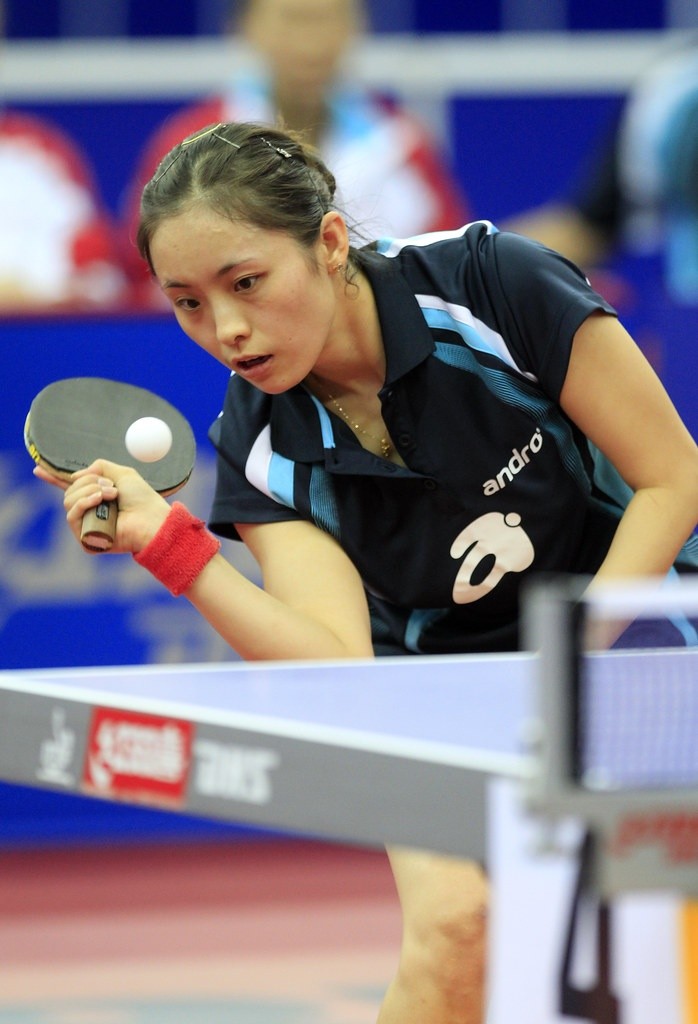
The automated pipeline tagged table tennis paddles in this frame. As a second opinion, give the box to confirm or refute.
[23,376,200,555]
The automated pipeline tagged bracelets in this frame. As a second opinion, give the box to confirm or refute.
[133,499,221,599]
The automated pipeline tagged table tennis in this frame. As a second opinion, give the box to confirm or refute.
[123,414,174,464]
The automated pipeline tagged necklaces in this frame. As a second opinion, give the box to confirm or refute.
[328,390,392,458]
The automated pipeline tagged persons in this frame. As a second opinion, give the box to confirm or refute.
[33,122,697,1023]
[0,0,698,312]
